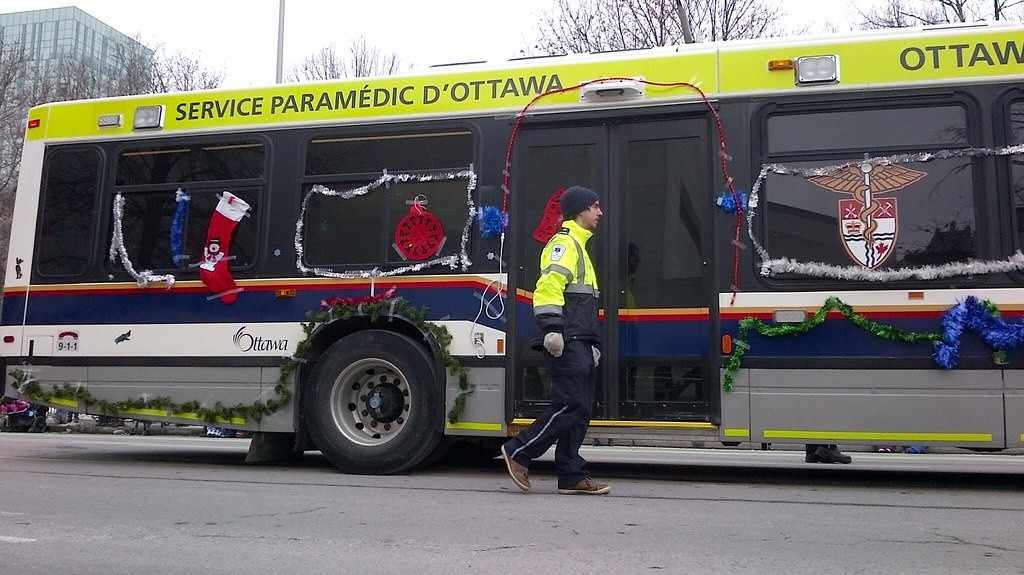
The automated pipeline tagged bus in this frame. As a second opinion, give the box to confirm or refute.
[0,20,1024,476]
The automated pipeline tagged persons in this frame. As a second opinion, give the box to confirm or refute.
[805,443,850,464]
[501,186,612,494]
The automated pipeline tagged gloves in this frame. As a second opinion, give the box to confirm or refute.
[591,345,601,367]
[543,332,564,357]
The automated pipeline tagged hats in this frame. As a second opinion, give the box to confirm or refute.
[559,187,599,221]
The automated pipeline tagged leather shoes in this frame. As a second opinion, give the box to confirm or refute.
[805,450,852,464]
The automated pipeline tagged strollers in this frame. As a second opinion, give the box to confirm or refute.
[0,397,48,434]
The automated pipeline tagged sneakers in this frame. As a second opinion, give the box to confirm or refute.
[501,442,532,490]
[557,478,612,495]
[878,448,891,453]
